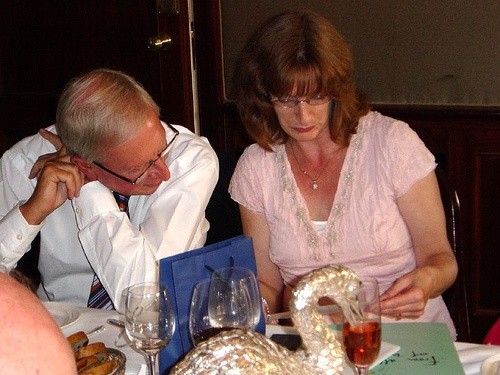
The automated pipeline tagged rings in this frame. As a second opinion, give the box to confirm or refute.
[397,313,403,319]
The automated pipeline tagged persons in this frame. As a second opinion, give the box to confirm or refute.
[0,272,78,375]
[0,70,222,314]
[230,8,461,342]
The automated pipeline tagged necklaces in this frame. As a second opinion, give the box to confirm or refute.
[287,137,332,191]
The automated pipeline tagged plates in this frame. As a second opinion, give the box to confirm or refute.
[481,354,500,375]
[41,300,82,330]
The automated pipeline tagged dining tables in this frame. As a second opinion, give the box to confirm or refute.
[59,307,500,375]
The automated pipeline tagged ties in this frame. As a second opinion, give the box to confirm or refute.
[86,192,131,312]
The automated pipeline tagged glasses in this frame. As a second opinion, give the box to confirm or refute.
[91,116,180,185]
[267,91,334,108]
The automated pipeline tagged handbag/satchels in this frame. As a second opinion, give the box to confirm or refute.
[155,234,266,375]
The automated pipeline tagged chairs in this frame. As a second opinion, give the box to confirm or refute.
[436,168,471,336]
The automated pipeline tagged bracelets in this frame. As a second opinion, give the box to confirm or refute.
[261,295,271,322]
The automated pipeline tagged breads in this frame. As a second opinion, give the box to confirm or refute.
[66,331,116,375]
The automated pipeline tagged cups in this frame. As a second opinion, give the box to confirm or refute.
[207,267,262,329]
[188,277,255,348]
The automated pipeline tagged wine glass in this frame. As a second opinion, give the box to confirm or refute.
[342,276,382,375]
[125,281,177,375]
